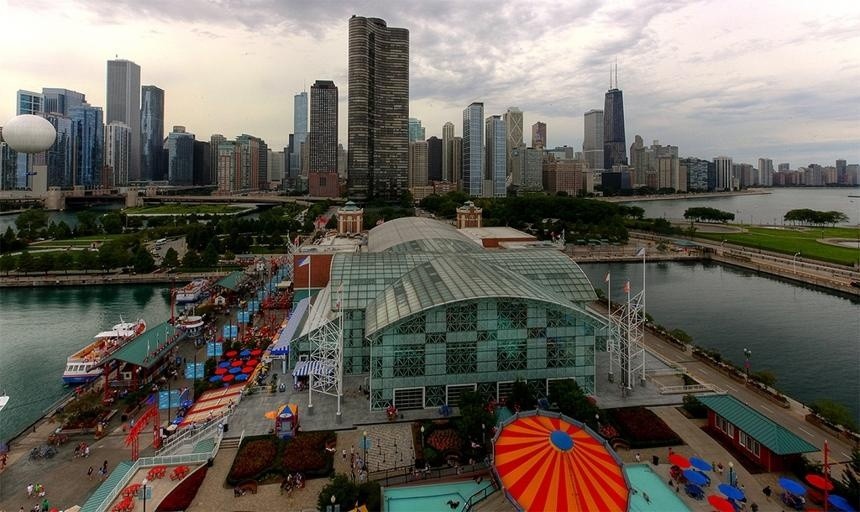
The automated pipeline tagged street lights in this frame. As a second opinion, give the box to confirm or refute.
[721,238,727,254]
[794,252,801,274]
[742,347,751,376]
[420,424,427,446]
[362,430,368,469]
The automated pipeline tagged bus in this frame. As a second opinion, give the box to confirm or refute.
[157,238,166,245]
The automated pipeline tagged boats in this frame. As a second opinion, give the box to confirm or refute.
[62,318,147,385]
[176,279,211,304]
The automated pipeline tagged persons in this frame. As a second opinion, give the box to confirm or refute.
[120,411,137,431]
[712,460,724,475]
[763,486,771,499]
[19,482,51,512]
[739,484,745,495]
[636,453,641,462]
[0,453,7,469]
[74,442,90,458]
[408,464,432,481]
[286,472,303,497]
[798,495,806,504]
[342,441,371,482]
[189,421,196,437]
[171,369,178,380]
[222,413,229,432]
[88,460,108,482]
[750,502,759,512]
